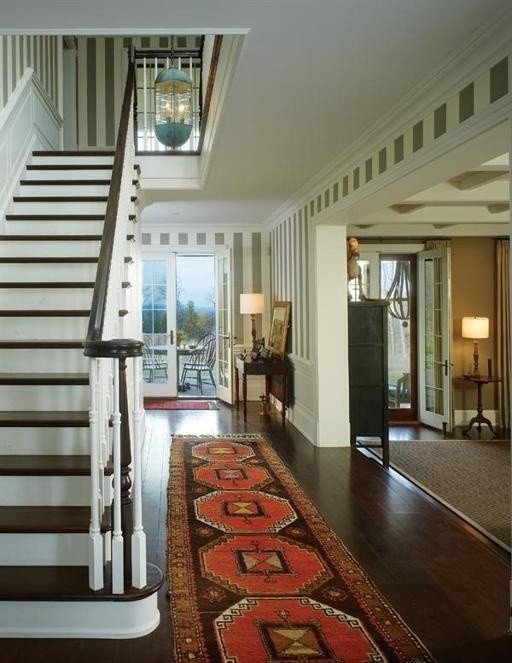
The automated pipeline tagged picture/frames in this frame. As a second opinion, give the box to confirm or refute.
[265,300,292,359]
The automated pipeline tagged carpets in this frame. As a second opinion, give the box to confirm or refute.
[162,430,435,661]
[365,436,510,556]
[142,400,220,411]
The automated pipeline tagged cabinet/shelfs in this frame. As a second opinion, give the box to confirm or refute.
[348,299,393,470]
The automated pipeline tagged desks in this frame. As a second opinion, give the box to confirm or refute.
[455,374,502,438]
[148,344,207,390]
[233,354,290,426]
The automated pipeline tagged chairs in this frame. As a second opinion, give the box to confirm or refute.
[179,331,218,394]
[142,334,168,383]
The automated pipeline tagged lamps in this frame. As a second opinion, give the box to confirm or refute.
[152,35,194,152]
[239,292,264,352]
[461,314,490,378]
